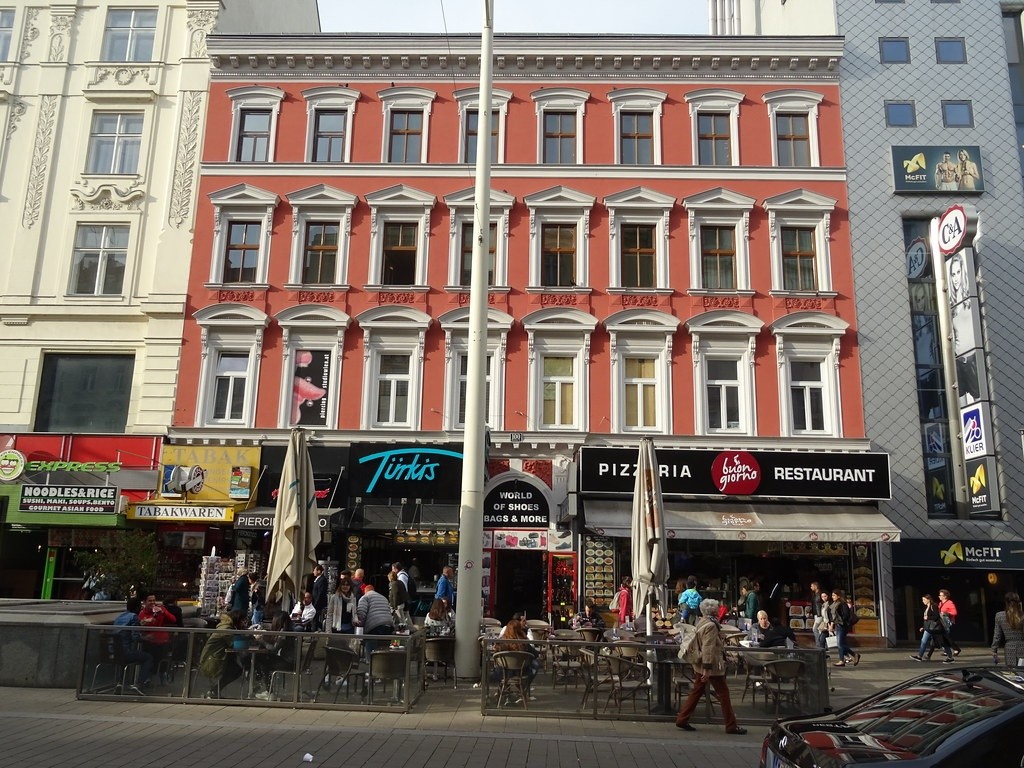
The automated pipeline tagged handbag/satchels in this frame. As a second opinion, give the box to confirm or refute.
[928,621,943,633]
[847,613,859,626]
[940,616,952,632]
[825,631,838,648]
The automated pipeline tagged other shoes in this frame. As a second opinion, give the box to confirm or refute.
[909,655,922,662]
[833,661,845,666]
[676,724,696,731]
[325,674,330,683]
[206,691,222,699]
[853,653,861,666]
[942,659,955,664]
[335,676,350,686]
[727,727,748,735]
[131,684,148,696]
[365,678,381,684]
[940,653,948,656]
[844,656,852,663]
[922,655,930,661]
[113,682,123,695]
[952,648,961,657]
[255,691,274,701]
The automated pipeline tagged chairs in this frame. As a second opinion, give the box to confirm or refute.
[483,618,807,718]
[90,616,457,706]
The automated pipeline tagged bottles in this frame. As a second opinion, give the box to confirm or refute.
[430,621,435,637]
[425,623,430,638]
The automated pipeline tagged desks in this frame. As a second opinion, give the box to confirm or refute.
[374,646,421,702]
[748,652,802,715]
[225,646,269,699]
[487,648,546,697]
[620,635,674,643]
[720,629,748,674]
[640,652,690,715]
[549,637,586,685]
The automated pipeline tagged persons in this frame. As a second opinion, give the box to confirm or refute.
[113,563,1024,735]
[829,590,861,666]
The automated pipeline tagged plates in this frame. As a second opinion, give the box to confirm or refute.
[789,605,804,616]
[805,606,814,618]
[806,619,815,629]
[586,541,614,606]
[789,619,805,629]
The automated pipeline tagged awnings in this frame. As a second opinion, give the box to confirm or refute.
[233,506,344,531]
[583,500,902,541]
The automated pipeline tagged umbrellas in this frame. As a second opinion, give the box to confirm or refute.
[631,433,670,688]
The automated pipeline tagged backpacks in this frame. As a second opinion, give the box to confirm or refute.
[608,590,629,610]
[399,571,417,601]
[677,619,714,664]
